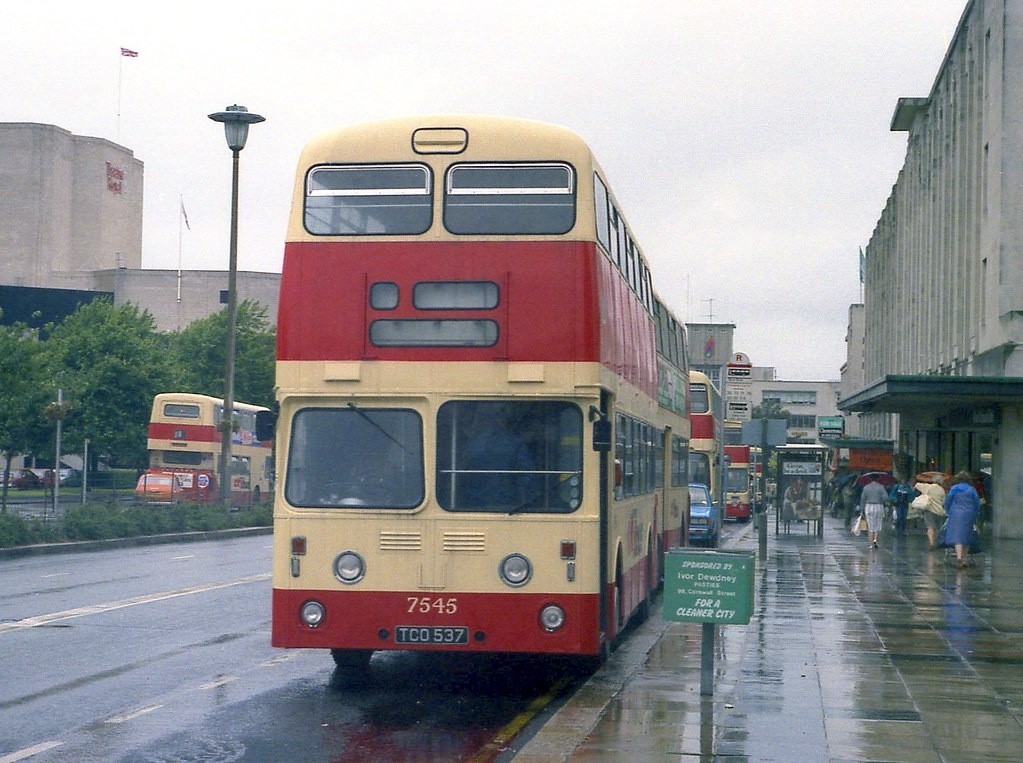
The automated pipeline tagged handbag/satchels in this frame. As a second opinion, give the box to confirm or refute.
[911,485,931,510]
[860,516,868,531]
[893,506,898,519]
[851,515,861,536]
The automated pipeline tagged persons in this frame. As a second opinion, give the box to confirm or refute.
[942,470,979,566]
[842,481,855,531]
[783,477,807,519]
[890,478,915,535]
[909,480,920,497]
[825,483,835,506]
[136,467,144,483]
[859,475,888,551]
[451,414,537,500]
[915,476,946,549]
[975,480,985,534]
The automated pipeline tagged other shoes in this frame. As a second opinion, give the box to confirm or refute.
[928,543,936,551]
[869,546,873,549]
[958,559,963,569]
[963,559,966,567]
[873,540,878,547]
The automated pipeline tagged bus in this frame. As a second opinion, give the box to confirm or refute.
[253,114,692,686]
[145,391,276,512]
[751,446,764,512]
[723,443,751,522]
[688,368,729,547]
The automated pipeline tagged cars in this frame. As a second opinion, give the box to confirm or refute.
[0,468,40,490]
[133,474,185,507]
[29,468,62,488]
[688,482,720,549]
[52,468,84,488]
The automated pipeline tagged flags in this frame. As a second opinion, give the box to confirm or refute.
[121,48,138,57]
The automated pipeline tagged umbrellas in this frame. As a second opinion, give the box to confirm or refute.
[916,472,949,483]
[859,471,896,486]
[837,474,858,491]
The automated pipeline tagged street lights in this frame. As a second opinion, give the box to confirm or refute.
[207,105,266,511]
